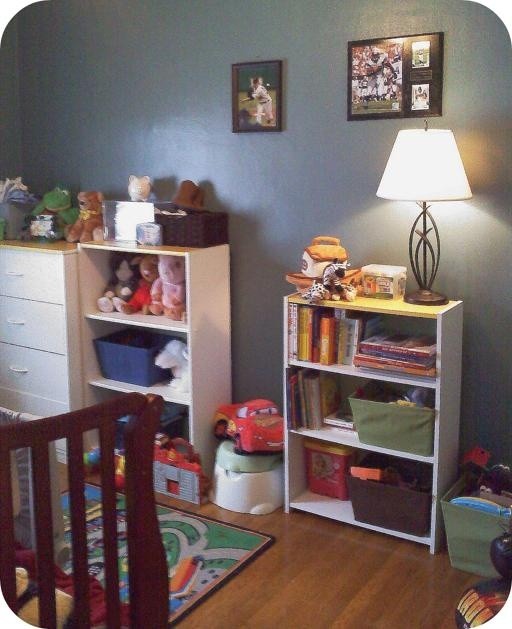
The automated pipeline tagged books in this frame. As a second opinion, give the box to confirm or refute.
[288,302,437,431]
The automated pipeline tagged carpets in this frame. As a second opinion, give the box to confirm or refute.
[43,478,275,623]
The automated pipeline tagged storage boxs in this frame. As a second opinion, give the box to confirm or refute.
[87,329,178,387]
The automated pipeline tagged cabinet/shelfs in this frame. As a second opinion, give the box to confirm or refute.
[76,237,232,484]
[1,248,93,464]
[280,288,466,555]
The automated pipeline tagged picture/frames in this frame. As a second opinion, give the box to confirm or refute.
[230,60,282,132]
[347,31,445,118]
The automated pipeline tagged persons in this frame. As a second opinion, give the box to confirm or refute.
[351,46,402,106]
[238,77,274,123]
[414,86,427,99]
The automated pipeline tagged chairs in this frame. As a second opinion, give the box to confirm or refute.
[1,391,174,629]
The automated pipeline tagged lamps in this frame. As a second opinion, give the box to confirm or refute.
[374,120,475,305]
